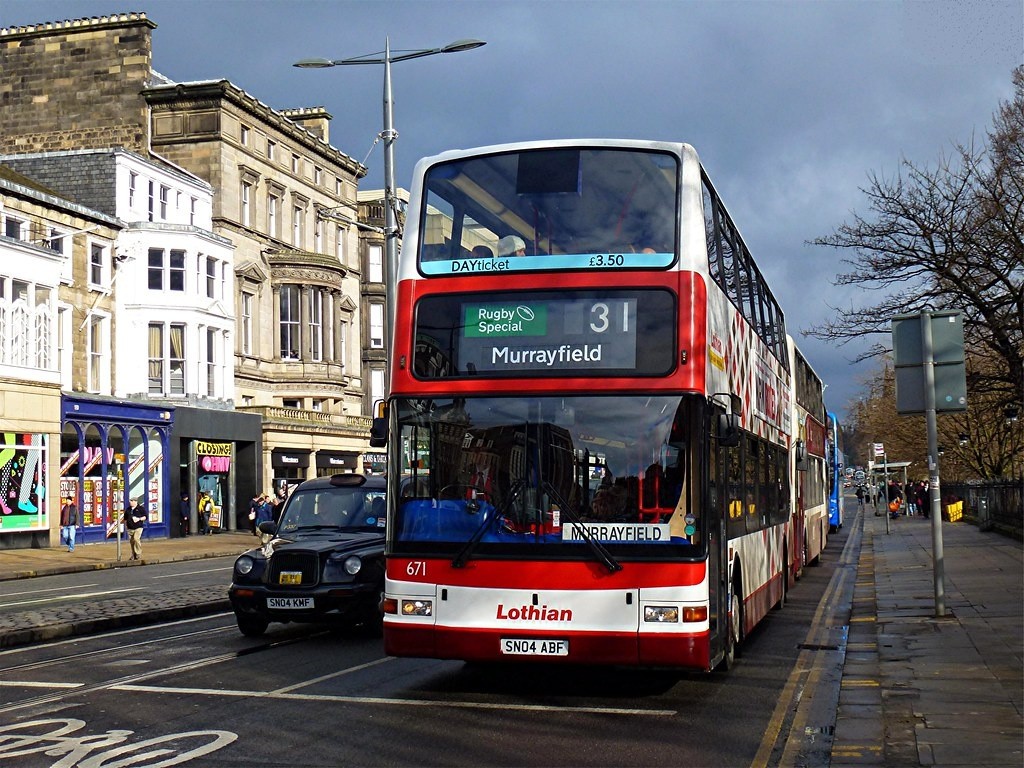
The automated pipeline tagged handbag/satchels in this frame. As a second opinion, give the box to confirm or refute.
[249,508,256,520]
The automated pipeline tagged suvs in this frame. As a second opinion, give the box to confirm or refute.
[229,472,389,639]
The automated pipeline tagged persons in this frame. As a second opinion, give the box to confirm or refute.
[197,490,215,536]
[855,478,931,519]
[578,463,678,523]
[495,235,530,258]
[179,494,191,537]
[60,495,80,554]
[312,494,344,530]
[364,495,387,530]
[456,446,511,509]
[249,491,274,538]
[123,497,149,561]
[470,244,495,259]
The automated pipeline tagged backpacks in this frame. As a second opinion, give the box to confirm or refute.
[203,497,211,512]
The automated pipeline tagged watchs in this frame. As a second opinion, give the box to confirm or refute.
[139,517,142,522]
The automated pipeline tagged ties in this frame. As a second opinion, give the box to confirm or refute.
[476,471,485,501]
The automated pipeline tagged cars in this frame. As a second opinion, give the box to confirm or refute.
[845,480,851,486]
[853,481,861,487]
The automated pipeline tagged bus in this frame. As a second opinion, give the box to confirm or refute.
[369,138,846,679]
[855,471,865,480]
[846,468,853,478]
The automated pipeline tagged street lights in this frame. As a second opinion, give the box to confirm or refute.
[292,39,487,492]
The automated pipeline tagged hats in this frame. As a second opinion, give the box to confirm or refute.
[130,496,137,501]
[66,496,72,501]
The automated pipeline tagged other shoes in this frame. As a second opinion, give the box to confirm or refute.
[923,514,930,519]
[68,550,74,552]
[910,512,913,516]
[209,530,213,536]
[130,554,135,561]
[205,532,208,535]
[135,554,142,560]
